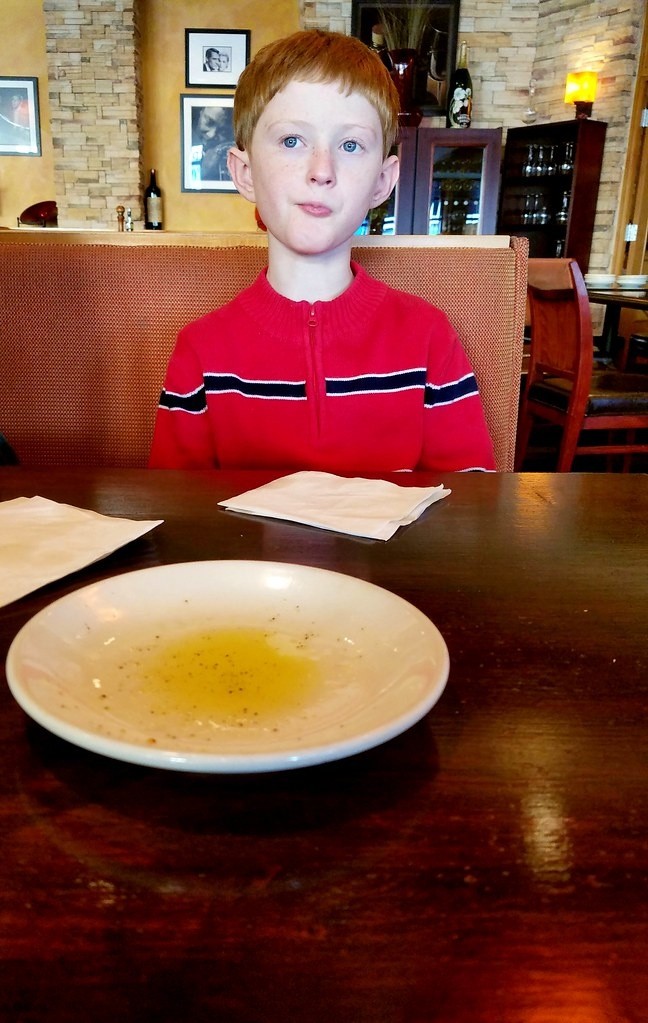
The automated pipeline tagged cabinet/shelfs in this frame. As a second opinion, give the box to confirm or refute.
[358,129,501,235]
[496,120,608,280]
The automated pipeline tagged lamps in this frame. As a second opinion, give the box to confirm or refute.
[565,72,598,119]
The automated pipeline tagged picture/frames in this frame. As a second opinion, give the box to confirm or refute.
[180,93,239,193]
[351,0,459,128]
[185,28,250,89]
[0,75,41,157]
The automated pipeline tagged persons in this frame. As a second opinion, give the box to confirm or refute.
[219,53,229,72]
[145,30,499,475]
[10,94,27,129]
[203,47,219,72]
[195,107,232,151]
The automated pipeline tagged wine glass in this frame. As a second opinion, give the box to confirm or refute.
[515,141,576,260]
[356,142,481,232]
[420,8,449,106]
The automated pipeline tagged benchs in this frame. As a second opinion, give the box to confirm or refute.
[1,230,529,469]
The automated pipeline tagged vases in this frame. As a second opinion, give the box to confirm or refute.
[387,49,422,127]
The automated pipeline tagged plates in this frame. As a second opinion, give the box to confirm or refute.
[584,272,648,287]
[7,559,449,773]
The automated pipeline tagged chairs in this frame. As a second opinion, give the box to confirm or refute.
[512,259,647,474]
[626,330,647,372]
[522,344,607,374]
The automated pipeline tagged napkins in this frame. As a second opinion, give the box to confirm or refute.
[217,469,452,541]
[1,496,163,607]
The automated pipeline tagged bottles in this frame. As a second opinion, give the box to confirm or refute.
[125,208,134,231]
[369,25,387,62]
[144,168,163,230]
[444,41,474,128]
[116,206,124,232]
[520,78,539,123]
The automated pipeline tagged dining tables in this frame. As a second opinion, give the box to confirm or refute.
[0,472,648,1021]
[585,274,648,355]
